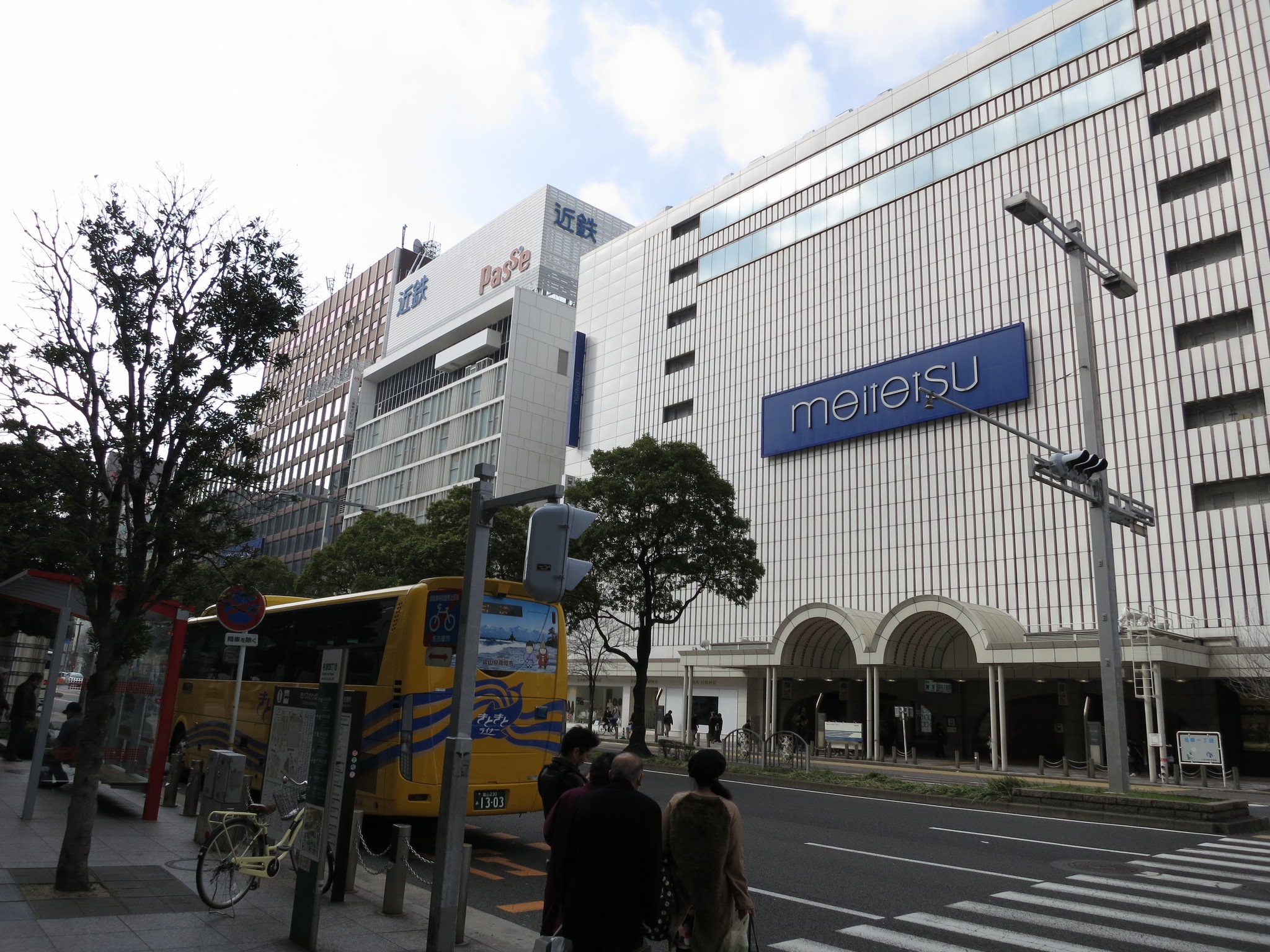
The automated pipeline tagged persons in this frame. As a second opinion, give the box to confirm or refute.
[42,701,81,787]
[6,672,44,761]
[538,706,756,952]
[606,699,613,711]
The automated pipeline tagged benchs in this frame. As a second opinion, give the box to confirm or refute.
[63,758,149,790]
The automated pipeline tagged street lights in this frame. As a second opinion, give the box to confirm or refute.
[1006,190,1138,794]
[276,487,379,549]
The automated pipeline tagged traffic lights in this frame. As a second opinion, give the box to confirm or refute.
[1048,449,1108,483]
[523,503,599,604]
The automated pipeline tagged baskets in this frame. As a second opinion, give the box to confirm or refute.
[272,782,307,820]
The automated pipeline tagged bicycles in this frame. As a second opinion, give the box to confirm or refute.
[195,773,335,919]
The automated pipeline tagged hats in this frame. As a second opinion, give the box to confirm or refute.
[0,666,10,673]
[62,702,82,714]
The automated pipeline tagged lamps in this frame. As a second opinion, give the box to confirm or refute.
[1057,628,1071,631]
[1118,607,1170,630]
[700,640,710,651]
[741,639,750,642]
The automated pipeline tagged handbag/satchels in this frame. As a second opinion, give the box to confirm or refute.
[532,923,572,952]
[720,912,750,952]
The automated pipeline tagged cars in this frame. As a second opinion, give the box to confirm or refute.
[40,668,68,689]
[64,672,84,687]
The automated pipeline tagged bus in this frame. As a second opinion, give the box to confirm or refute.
[168,578,570,856]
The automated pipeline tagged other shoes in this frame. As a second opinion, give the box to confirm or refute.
[52,780,69,787]
[7,756,22,761]
